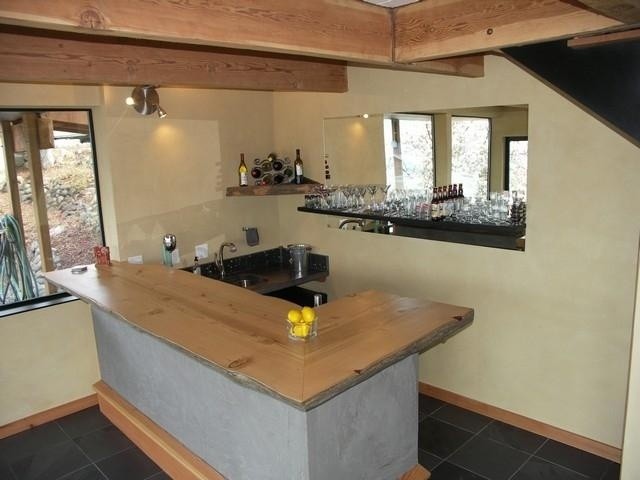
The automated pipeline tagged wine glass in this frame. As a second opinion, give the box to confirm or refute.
[465,191,525,224]
[341,184,434,218]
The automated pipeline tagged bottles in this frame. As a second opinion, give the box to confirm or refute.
[294,148,304,184]
[430,183,464,221]
[239,153,248,187]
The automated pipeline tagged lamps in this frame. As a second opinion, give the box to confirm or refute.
[125,85,168,119]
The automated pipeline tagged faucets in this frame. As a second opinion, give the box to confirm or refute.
[338,218,368,231]
[212,241,238,279]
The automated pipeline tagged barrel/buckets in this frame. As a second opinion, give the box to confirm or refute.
[287,242,312,272]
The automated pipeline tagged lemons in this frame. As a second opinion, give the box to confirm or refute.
[287,306,315,338]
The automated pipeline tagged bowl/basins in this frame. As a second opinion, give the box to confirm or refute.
[287,319,319,341]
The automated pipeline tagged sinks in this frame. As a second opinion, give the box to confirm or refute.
[222,275,265,289]
